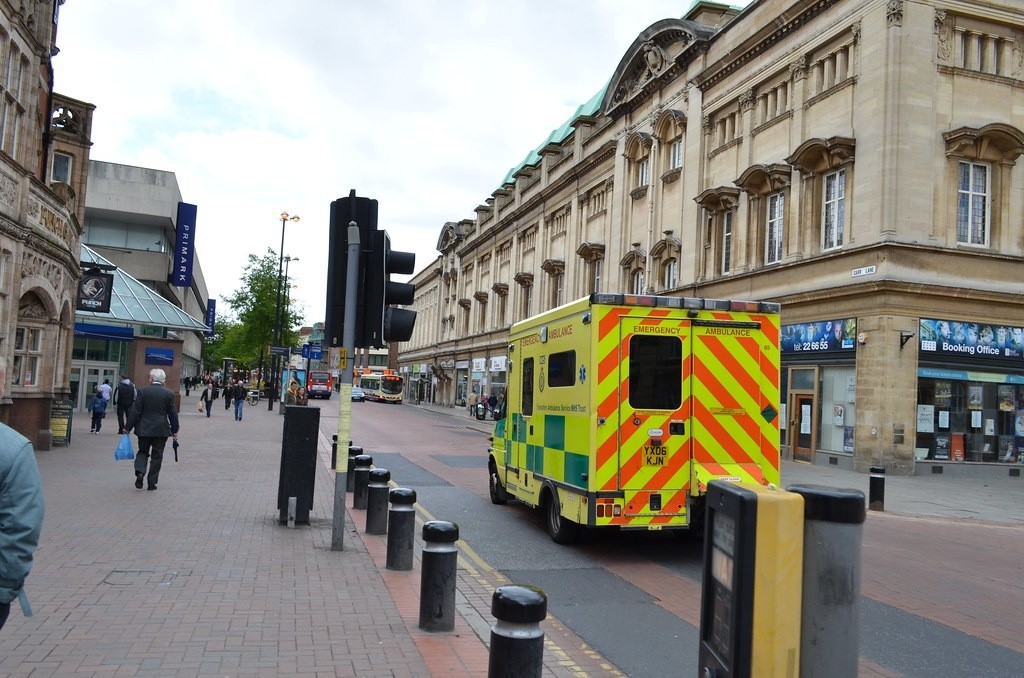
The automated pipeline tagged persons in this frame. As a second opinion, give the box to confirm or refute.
[834,323,844,340]
[233,380,247,421]
[284,371,301,391]
[123,368,180,490]
[98,378,112,418]
[200,383,217,418]
[296,386,309,406]
[88,388,108,434]
[0,423,45,628]
[488,393,498,417]
[112,372,137,434]
[214,382,221,398]
[222,382,233,409]
[284,381,297,412]
[481,393,488,412]
[497,393,504,413]
[185,378,194,396]
[469,390,478,416]
[200,375,203,386]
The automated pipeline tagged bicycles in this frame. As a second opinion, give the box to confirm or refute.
[231,389,259,406]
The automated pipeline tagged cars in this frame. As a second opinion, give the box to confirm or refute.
[351,386,366,402]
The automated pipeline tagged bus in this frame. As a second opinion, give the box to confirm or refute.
[359,368,406,405]
[306,370,334,400]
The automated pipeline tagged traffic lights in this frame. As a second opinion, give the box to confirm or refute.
[373,228,418,351]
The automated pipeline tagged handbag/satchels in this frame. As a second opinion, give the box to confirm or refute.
[198,401,204,412]
[114,433,135,461]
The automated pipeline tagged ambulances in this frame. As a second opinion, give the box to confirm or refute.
[473,291,783,546]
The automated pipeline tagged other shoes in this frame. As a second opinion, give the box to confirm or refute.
[135,473,143,489]
[148,484,157,490]
[90,428,95,433]
[239,418,242,421]
[207,415,210,417]
[235,417,238,420]
[95,432,100,435]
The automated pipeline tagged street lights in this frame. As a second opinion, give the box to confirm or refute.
[267,211,301,411]
